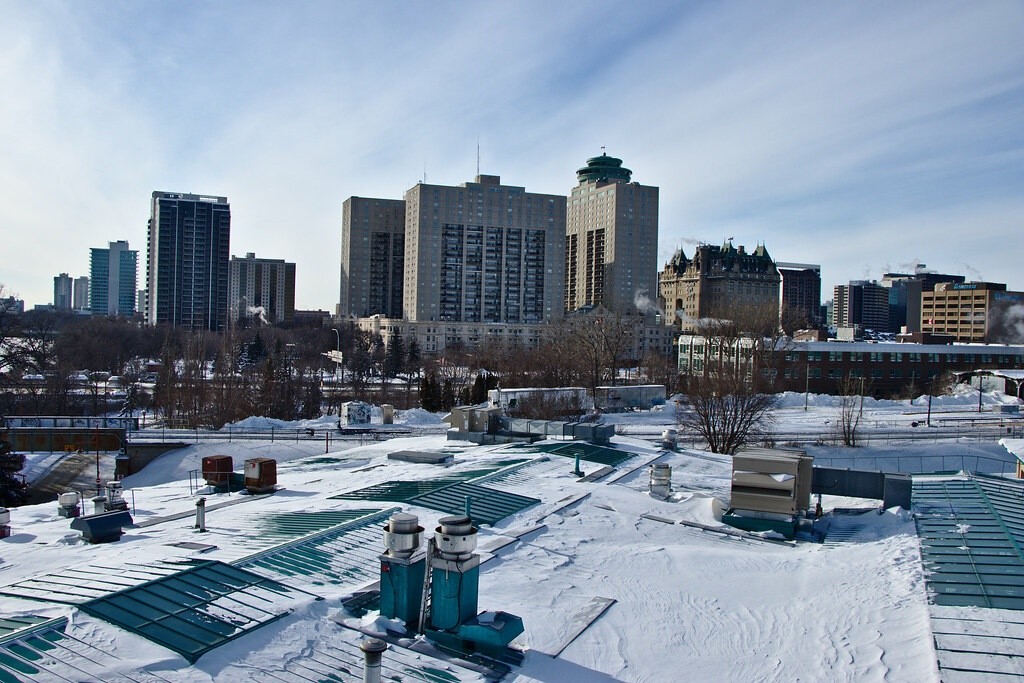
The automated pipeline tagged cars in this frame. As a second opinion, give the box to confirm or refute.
[22,369,157,383]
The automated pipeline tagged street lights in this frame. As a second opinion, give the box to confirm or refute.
[332,327,339,393]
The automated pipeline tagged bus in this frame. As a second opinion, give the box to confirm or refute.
[486,387,588,415]
[595,384,667,413]
